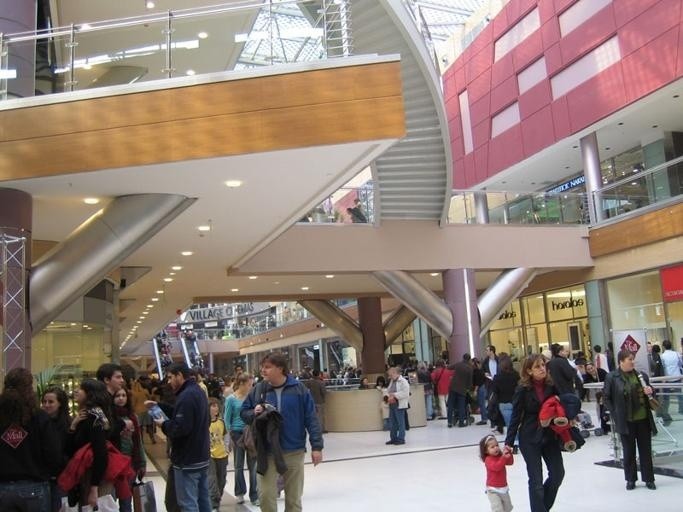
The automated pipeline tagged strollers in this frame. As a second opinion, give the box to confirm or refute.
[574,409,605,439]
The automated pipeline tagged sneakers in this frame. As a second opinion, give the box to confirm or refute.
[393,441,405,445]
[213,507,219,512]
[646,481,656,490]
[385,440,395,445]
[236,494,244,504]
[251,498,261,506]
[426,413,489,428]
[626,480,635,490]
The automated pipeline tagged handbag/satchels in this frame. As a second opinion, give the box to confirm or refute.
[81,493,121,512]
[237,381,267,459]
[636,370,661,411]
[132,478,157,512]
[434,385,438,397]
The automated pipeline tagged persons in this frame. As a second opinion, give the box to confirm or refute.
[352,199,365,224]
